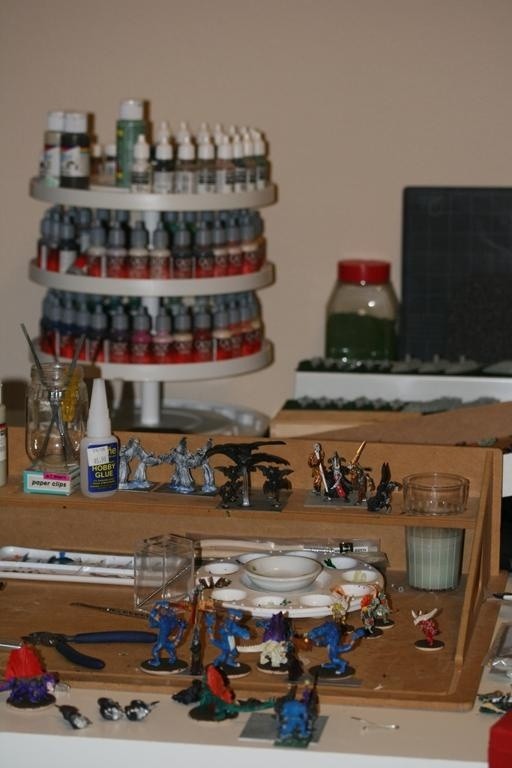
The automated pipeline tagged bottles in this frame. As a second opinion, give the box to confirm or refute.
[1,384,8,487]
[324,259,399,359]
[39,288,262,363]
[78,377,120,498]
[41,110,62,183]
[58,111,91,189]
[38,204,267,279]
[93,97,271,193]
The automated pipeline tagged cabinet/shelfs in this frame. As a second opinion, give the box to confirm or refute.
[0,407,502,713]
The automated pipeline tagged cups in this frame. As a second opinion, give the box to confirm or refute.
[135,533,194,614]
[24,361,89,471]
[403,472,470,592]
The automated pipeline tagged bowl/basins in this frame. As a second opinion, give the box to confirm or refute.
[241,556,323,590]
